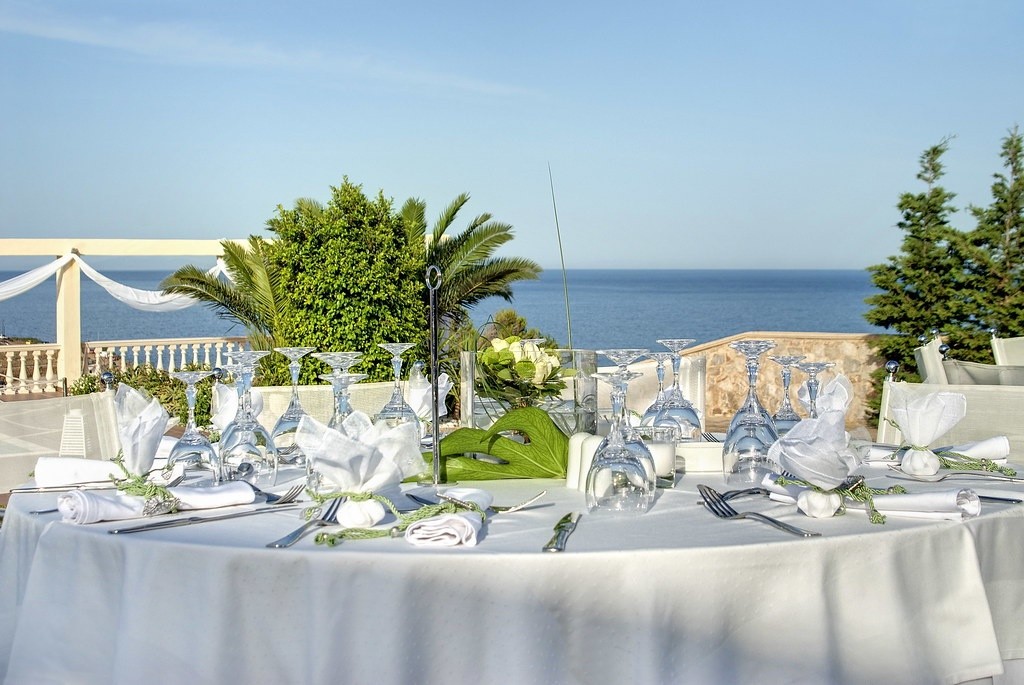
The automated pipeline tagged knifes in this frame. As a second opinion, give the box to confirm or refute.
[542,511,581,552]
[107,504,296,533]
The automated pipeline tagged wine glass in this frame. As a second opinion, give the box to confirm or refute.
[584,337,836,517]
[167,370,221,487]
[222,346,369,487]
[375,343,421,447]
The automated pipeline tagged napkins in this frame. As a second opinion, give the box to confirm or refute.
[32,377,1011,551]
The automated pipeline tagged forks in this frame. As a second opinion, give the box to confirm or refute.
[243,482,306,505]
[488,489,547,514]
[699,488,822,538]
[885,464,1024,484]
[265,497,348,547]
[165,475,186,486]
[701,432,725,442]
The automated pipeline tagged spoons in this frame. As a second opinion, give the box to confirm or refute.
[406,493,436,507]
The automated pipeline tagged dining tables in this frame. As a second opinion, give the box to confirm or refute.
[0,467,1024,685]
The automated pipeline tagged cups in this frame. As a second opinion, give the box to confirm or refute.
[634,426,676,488]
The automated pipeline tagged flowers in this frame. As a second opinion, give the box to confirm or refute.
[470,318,568,445]
